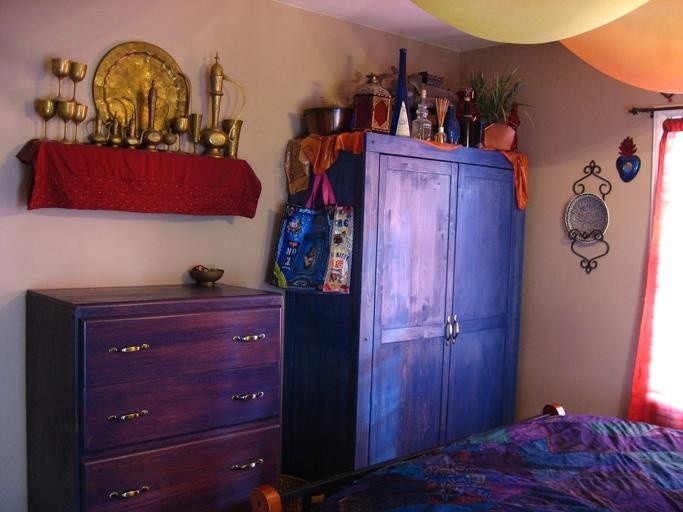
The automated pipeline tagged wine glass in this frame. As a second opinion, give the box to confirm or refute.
[36,57,87,144]
[189,113,203,155]
[172,118,189,154]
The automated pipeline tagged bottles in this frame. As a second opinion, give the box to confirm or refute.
[461,96,473,148]
[445,105,460,145]
[509,102,520,151]
[411,90,433,143]
[434,127,448,144]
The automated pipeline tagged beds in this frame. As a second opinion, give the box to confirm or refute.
[250,403,683,512]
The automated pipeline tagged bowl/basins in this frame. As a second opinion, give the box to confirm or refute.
[303,107,352,136]
[189,270,223,287]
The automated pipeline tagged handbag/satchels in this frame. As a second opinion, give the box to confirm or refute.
[269,172,354,295]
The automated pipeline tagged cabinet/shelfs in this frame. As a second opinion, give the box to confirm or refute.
[282,132,528,477]
[27,283,284,511]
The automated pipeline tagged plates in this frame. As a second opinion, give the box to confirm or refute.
[91,41,192,130]
[565,192,608,245]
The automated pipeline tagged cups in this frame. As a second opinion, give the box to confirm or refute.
[222,119,243,159]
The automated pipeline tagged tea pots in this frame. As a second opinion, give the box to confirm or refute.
[85,107,147,149]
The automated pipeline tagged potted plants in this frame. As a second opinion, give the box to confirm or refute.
[471,63,539,151]
